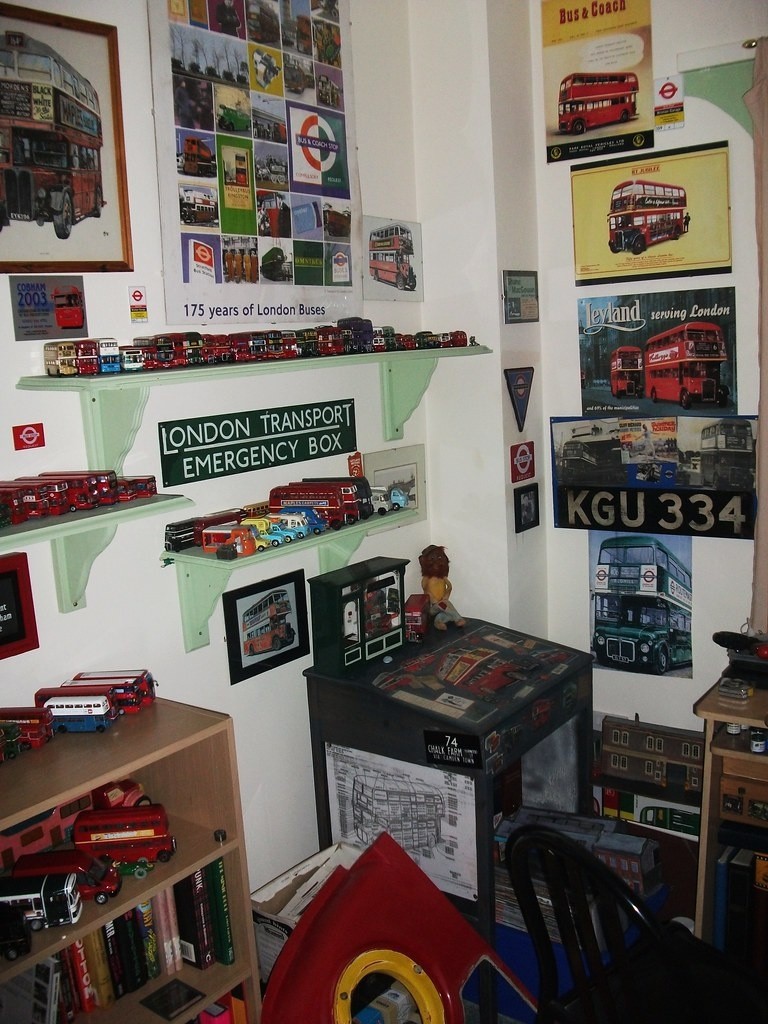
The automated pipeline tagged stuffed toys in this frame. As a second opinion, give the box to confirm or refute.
[418,544,466,630]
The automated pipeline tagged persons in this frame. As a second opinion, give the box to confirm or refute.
[216,0,241,38]
[225,247,258,284]
[637,423,659,457]
[174,79,214,131]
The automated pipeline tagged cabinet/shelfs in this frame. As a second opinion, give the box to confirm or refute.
[0,344,493,652]
[0,698,264,1024]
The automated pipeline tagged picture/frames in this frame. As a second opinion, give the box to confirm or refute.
[513,483,540,533]
[363,444,427,537]
[221,568,311,686]
[0,551,40,661]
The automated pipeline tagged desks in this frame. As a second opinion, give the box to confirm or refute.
[694,677,768,946]
[304,615,596,1024]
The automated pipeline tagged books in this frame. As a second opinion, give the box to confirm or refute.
[713,845,768,982]
[0,858,237,1024]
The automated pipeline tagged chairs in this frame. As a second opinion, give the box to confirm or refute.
[504,824,768,1024]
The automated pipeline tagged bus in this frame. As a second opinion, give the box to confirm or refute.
[606,180,691,255]
[698,418,757,492]
[592,535,693,677]
[562,439,603,487]
[610,346,644,400]
[402,593,432,649]
[0,669,160,766]
[557,72,640,136]
[368,224,418,292]
[242,588,297,658]
[0,469,157,528]
[644,321,730,410]
[1,772,177,960]
[162,476,409,559]
[0,26,99,241]
[43,314,467,378]
[173,3,355,290]
